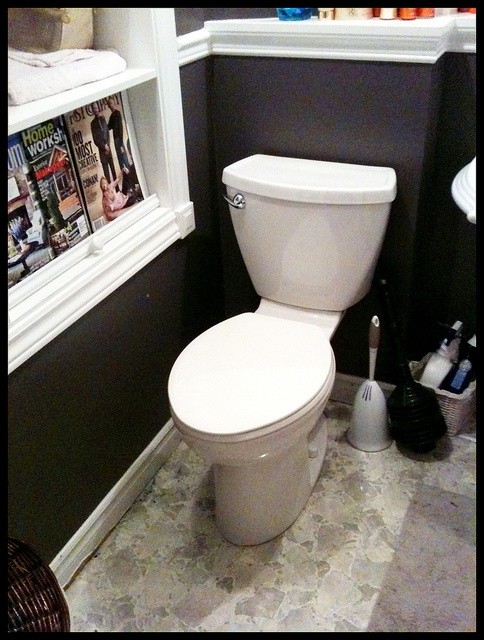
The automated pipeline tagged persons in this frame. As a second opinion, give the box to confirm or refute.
[89,101,119,193]
[106,96,130,171]
[121,137,143,201]
[98,168,142,212]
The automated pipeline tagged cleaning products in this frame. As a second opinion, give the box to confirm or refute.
[443,336,477,395]
[417,321,464,387]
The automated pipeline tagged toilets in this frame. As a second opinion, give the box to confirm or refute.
[167,152,399,547]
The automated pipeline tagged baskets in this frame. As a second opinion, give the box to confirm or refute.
[411,352,476,436]
[6,537,71,632]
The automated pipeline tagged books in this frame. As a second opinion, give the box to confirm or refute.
[19,117,91,256]
[63,92,146,235]
[8,133,56,288]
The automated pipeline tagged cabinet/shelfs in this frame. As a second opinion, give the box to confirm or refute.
[8,9,196,375]
